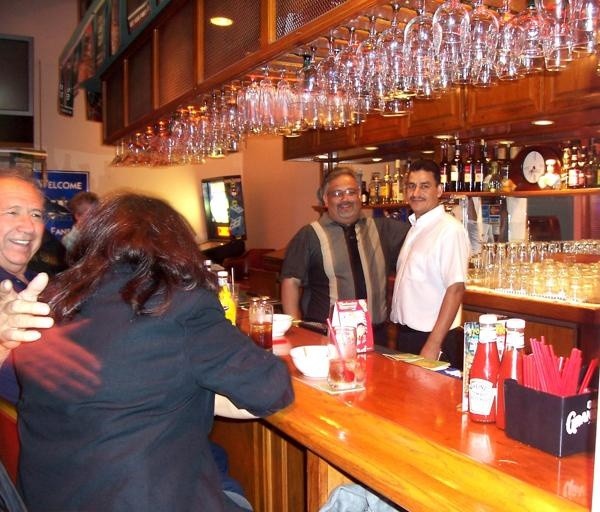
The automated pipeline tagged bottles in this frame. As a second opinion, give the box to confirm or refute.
[249,296,270,324]
[466,240,600,303]
[204,259,212,271]
[217,272,228,288]
[495,318,528,432]
[487,197,504,241]
[534,159,560,190]
[438,140,525,192]
[361,159,411,206]
[467,312,500,422]
[560,138,600,189]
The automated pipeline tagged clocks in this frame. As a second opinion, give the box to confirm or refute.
[507,146,563,192]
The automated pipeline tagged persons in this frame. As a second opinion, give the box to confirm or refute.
[61,192,98,253]
[390,159,469,371]
[16,194,294,512]
[0,167,54,485]
[282,168,410,349]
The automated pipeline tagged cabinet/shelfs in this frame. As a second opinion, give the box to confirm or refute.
[462,305,580,362]
[284,38,356,163]
[467,29,600,139]
[358,47,466,151]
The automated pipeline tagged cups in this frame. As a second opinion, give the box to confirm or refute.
[329,325,357,389]
[223,283,240,302]
[247,304,273,353]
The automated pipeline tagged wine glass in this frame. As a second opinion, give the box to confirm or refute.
[106,0,600,168]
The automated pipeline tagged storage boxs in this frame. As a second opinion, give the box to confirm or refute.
[502,369,597,457]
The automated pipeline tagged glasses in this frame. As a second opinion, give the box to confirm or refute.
[332,190,358,197]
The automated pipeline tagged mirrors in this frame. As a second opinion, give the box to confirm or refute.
[464,195,600,255]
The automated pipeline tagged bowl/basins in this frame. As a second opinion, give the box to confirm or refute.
[272,314,292,336]
[289,344,329,377]
